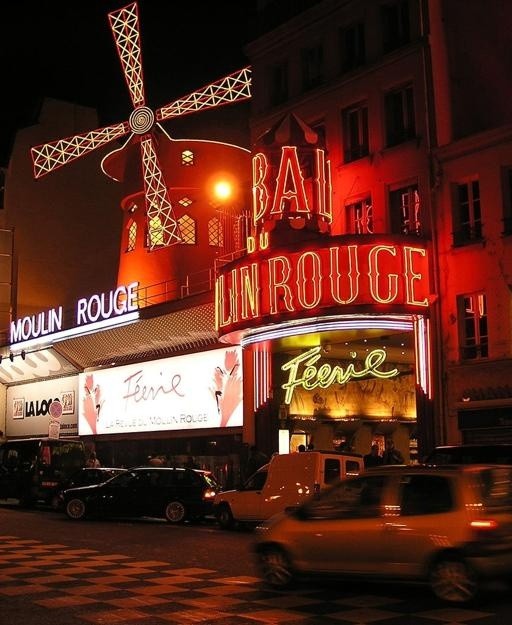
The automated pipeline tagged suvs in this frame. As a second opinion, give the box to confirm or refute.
[64,467,220,525]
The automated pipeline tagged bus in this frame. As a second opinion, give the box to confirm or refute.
[1,438,85,506]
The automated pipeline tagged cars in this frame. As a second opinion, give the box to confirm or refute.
[250,464,511,605]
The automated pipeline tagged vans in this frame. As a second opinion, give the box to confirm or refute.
[213,451,363,531]
[70,467,139,488]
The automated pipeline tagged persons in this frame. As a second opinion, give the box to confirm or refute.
[147,452,201,470]
[383,438,405,464]
[86,451,101,468]
[364,444,382,468]
